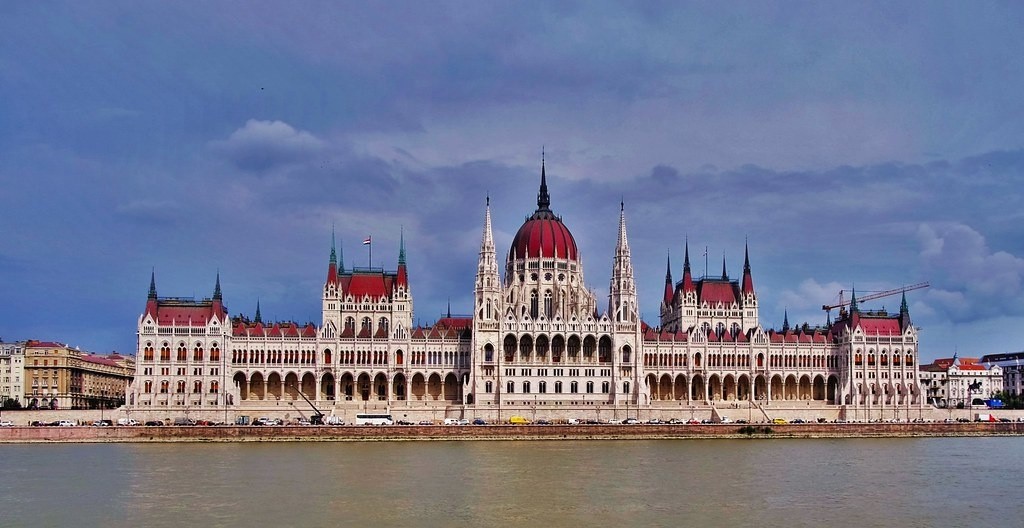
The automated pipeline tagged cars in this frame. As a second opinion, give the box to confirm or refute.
[93,420,108,426]
[773,418,788,424]
[154,421,163,426]
[419,420,434,425]
[0,420,14,426]
[538,416,749,425]
[31,421,43,427]
[793,414,1024,423]
[299,420,311,426]
[145,421,155,426]
[59,420,77,426]
[444,418,488,425]
[258,417,282,426]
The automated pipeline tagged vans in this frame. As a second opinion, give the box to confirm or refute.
[510,416,530,425]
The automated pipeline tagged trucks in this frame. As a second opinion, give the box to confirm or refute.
[118,418,138,425]
[174,416,195,426]
[327,416,345,425]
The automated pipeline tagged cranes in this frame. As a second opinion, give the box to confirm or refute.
[822,281,930,318]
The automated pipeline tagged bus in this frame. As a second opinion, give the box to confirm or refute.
[356,414,394,425]
